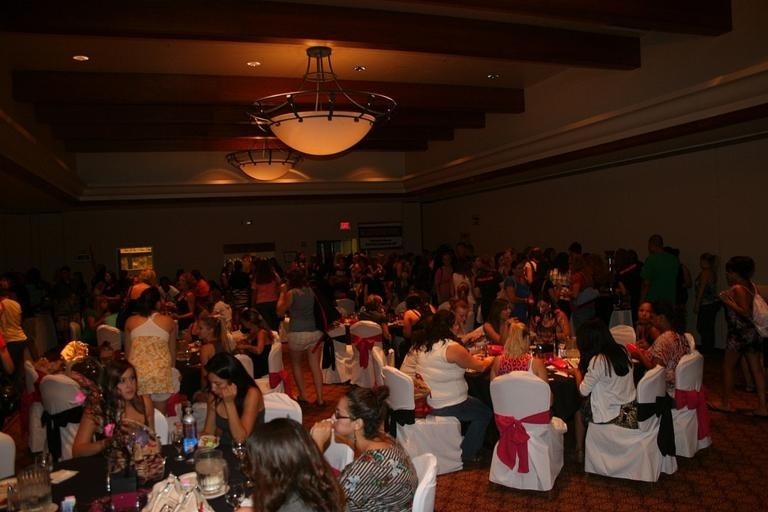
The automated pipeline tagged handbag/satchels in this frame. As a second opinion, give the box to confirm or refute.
[497,288,517,302]
[574,288,600,306]
[120,419,164,483]
[141,473,213,512]
[614,404,638,428]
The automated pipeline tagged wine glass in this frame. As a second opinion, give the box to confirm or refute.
[225,479,246,511]
[183,348,192,366]
[171,428,186,463]
[231,436,246,470]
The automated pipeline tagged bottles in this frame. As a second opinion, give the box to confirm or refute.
[16,470,52,511]
[183,408,198,438]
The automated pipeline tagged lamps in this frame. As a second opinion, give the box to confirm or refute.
[225,104,305,182]
[246,46,399,155]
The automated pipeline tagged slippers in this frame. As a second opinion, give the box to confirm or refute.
[317,400,325,407]
[743,410,768,418]
[706,400,729,411]
[298,396,307,402]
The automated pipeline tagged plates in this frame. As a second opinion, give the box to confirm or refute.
[196,482,230,500]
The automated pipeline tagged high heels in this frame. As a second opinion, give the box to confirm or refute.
[574,449,583,462]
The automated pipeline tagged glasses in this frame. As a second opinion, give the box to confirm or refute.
[335,409,350,419]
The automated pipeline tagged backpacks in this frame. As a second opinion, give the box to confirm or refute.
[745,283,768,338]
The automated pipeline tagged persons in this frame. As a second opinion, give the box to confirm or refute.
[1,231,767,512]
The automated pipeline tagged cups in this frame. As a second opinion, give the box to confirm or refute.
[37,453,51,469]
[194,447,228,494]
